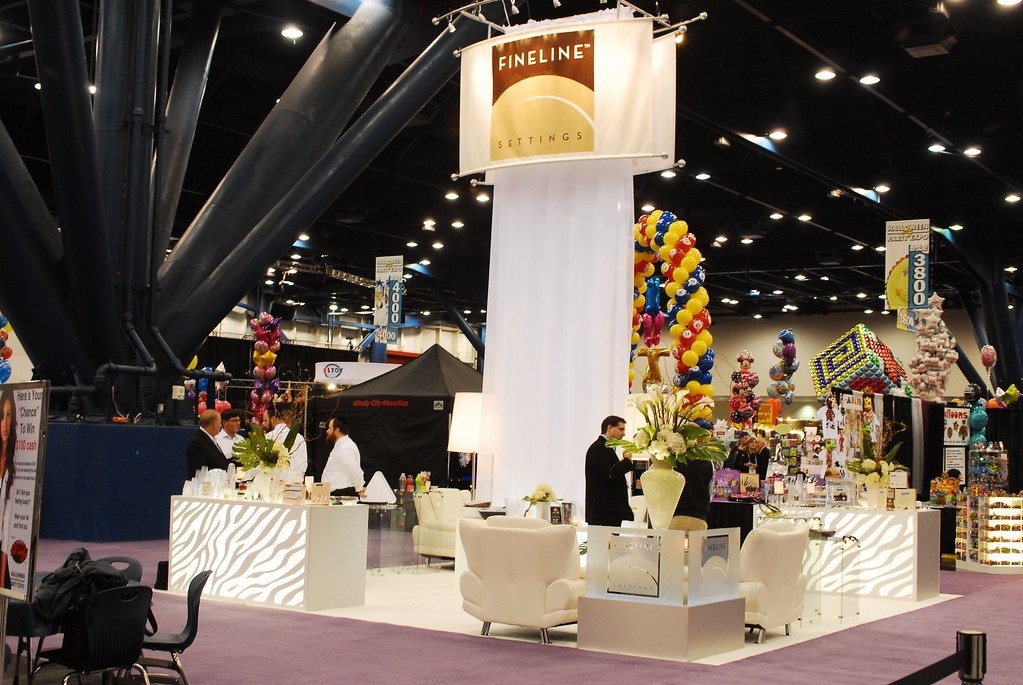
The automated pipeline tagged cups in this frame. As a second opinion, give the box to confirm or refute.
[181,463,235,498]
[431,486,438,491]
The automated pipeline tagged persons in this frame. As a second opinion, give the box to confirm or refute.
[320,419,367,501]
[584,415,634,536]
[186,408,248,479]
[947,469,961,482]
[0,389,18,591]
[723,430,770,480]
[262,408,308,484]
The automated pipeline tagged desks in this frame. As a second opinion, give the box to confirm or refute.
[5,572,145,685]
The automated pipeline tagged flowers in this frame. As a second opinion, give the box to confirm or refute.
[605,384,728,468]
[232,417,301,474]
[522,485,556,505]
[847,420,910,488]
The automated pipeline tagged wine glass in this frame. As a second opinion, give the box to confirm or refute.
[305,476,314,502]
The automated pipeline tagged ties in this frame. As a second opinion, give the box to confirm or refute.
[217,443,226,459]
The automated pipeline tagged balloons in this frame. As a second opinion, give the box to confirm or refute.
[968,435,987,451]
[628,209,715,432]
[964,383,988,430]
[249,311,282,431]
[730,353,762,430]
[767,328,800,405]
[986,384,1021,408]
[188,358,231,412]
[982,344,997,367]
[909,293,959,397]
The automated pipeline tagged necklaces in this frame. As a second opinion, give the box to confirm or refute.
[759,446,767,454]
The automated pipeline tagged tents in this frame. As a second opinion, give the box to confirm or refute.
[308,344,484,490]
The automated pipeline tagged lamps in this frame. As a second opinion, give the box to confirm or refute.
[431,0,607,32]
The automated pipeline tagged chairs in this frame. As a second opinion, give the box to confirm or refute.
[34,556,143,668]
[29,585,150,685]
[101,570,213,685]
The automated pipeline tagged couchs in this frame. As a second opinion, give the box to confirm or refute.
[412,487,471,567]
[739,520,810,645]
[460,516,587,644]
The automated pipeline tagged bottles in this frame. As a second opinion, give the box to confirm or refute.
[556,498,576,524]
[399,471,431,493]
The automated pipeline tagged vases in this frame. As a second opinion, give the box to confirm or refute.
[867,485,887,511]
[640,453,686,530]
[262,470,282,503]
[537,502,550,522]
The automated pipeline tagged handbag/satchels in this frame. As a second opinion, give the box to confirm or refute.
[60,593,158,662]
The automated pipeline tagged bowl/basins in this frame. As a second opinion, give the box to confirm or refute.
[330,496,358,505]
[480,509,506,519]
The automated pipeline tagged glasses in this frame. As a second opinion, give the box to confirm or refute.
[229,419,241,423]
